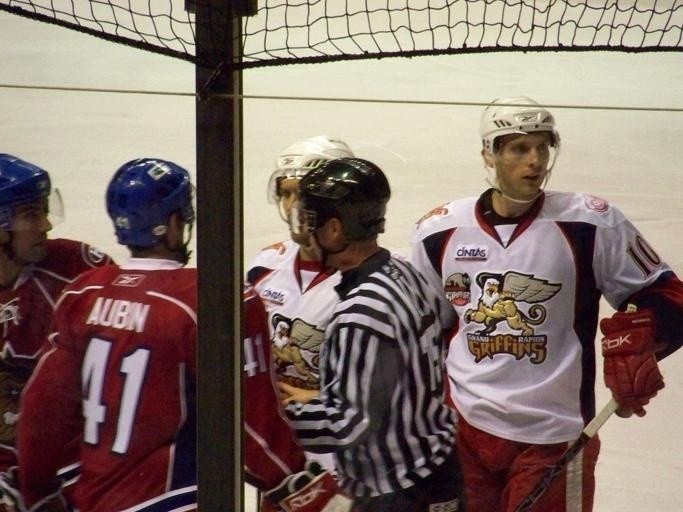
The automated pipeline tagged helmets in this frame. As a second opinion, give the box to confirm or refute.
[0,153,52,218]
[105,159,191,247]
[267,137,392,240]
[480,97,560,155]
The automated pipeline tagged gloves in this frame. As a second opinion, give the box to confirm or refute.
[600,312,664,418]
